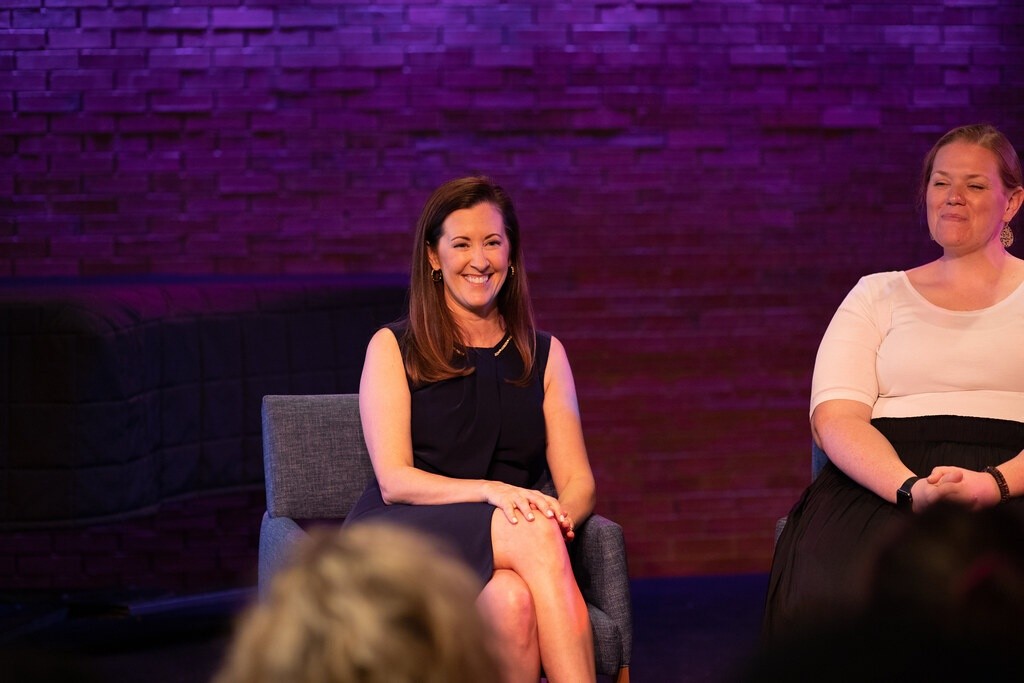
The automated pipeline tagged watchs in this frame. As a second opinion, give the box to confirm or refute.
[897,477,921,508]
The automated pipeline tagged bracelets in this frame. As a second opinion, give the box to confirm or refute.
[981,466,1010,503]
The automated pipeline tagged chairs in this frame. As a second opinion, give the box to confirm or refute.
[258,391,637,683]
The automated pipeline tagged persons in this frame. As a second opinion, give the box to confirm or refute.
[341,177,597,683]
[217,520,504,683]
[764,124,1024,638]
